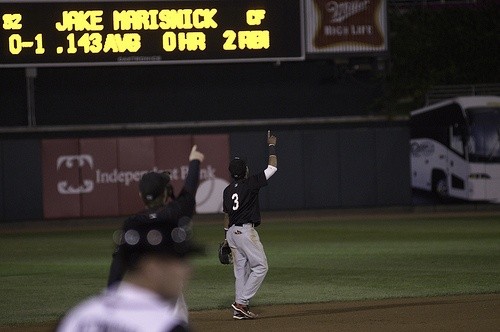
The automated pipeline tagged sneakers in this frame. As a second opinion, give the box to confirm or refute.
[232,310,250,320]
[231,302,255,319]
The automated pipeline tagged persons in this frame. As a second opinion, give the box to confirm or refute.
[58,145,206,332]
[223,130,279,319]
[335,0,500,114]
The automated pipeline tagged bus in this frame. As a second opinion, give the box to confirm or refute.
[409,94,496,206]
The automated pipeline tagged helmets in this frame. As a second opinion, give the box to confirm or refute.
[120,212,205,256]
[228,156,246,180]
[139,171,171,203]
